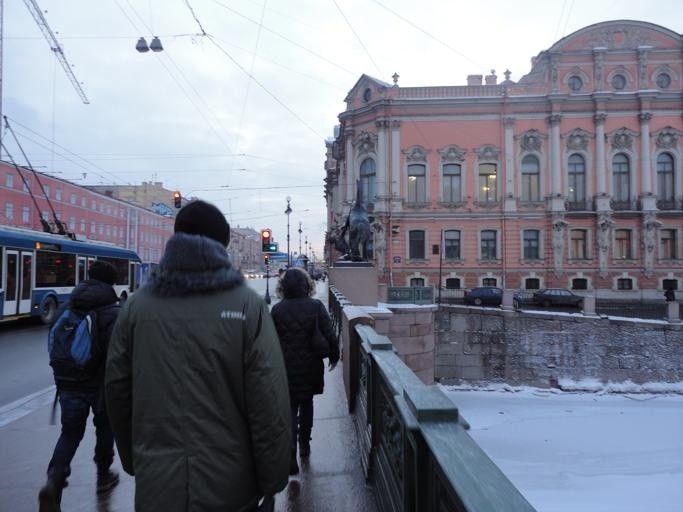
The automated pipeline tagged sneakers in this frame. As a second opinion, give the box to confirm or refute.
[97,470,119,492]
[39,480,61,512]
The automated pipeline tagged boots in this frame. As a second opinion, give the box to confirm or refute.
[289,433,299,475]
[298,428,312,457]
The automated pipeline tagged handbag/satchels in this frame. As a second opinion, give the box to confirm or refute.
[311,328,330,363]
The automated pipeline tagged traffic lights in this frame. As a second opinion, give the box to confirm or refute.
[264,256,268,265]
[174,191,182,209]
[260,228,278,254]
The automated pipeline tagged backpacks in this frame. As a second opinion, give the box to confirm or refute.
[47,302,121,384]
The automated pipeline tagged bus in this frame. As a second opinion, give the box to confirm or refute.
[0,222,147,328]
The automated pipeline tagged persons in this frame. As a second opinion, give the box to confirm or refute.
[37,255,123,511]
[269,263,340,477]
[103,201,298,511]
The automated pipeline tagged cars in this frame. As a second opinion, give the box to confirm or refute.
[532,288,587,309]
[462,286,522,307]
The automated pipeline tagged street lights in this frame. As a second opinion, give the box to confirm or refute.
[283,196,319,278]
